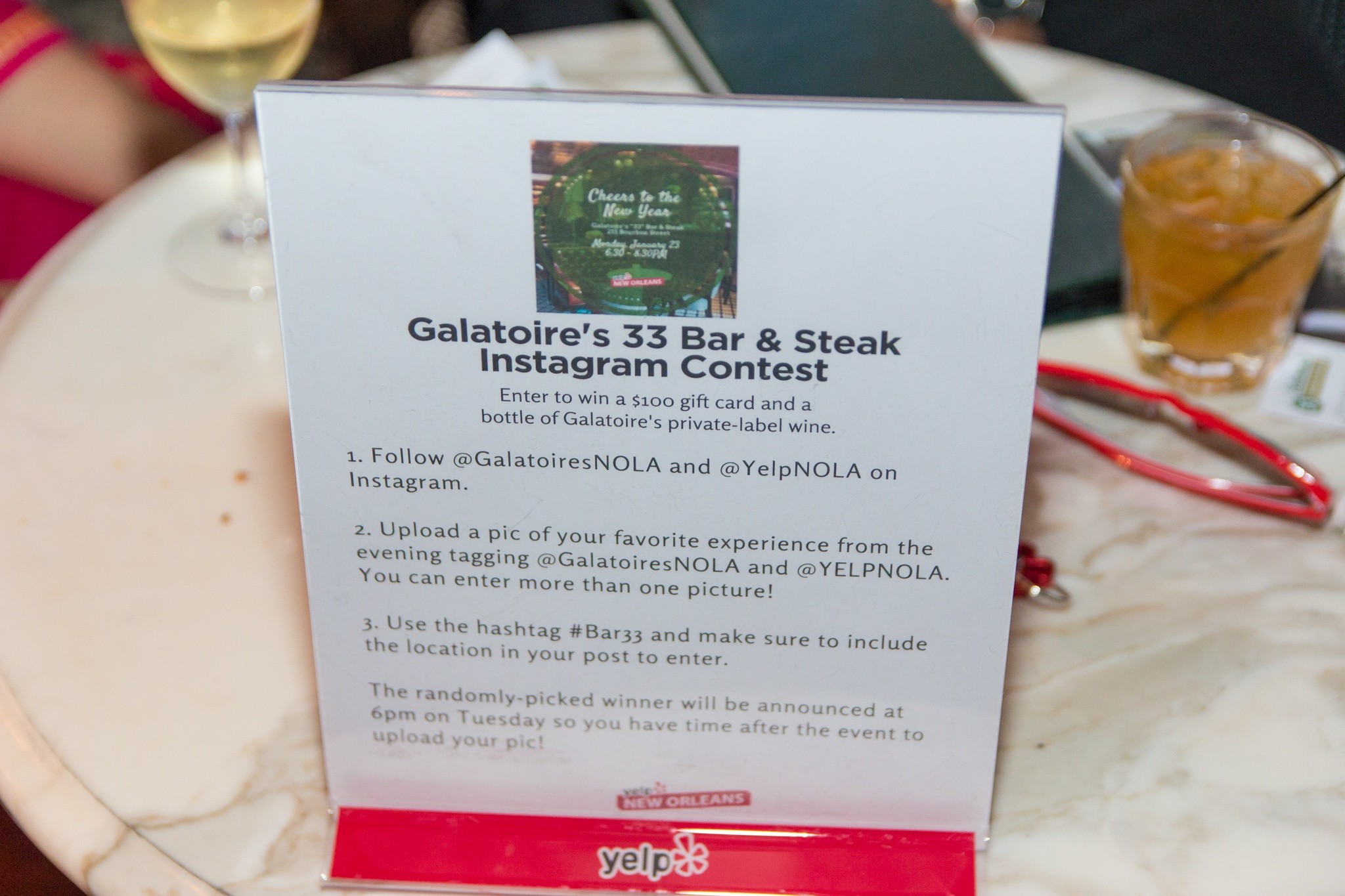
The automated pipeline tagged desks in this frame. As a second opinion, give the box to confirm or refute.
[0,24,1345,896]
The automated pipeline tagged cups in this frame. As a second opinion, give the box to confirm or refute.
[1116,110,1345,394]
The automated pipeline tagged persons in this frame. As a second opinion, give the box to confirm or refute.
[0,0,465,281]
[936,0,1345,154]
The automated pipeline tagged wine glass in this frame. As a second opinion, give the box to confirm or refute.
[118,0,326,304]
